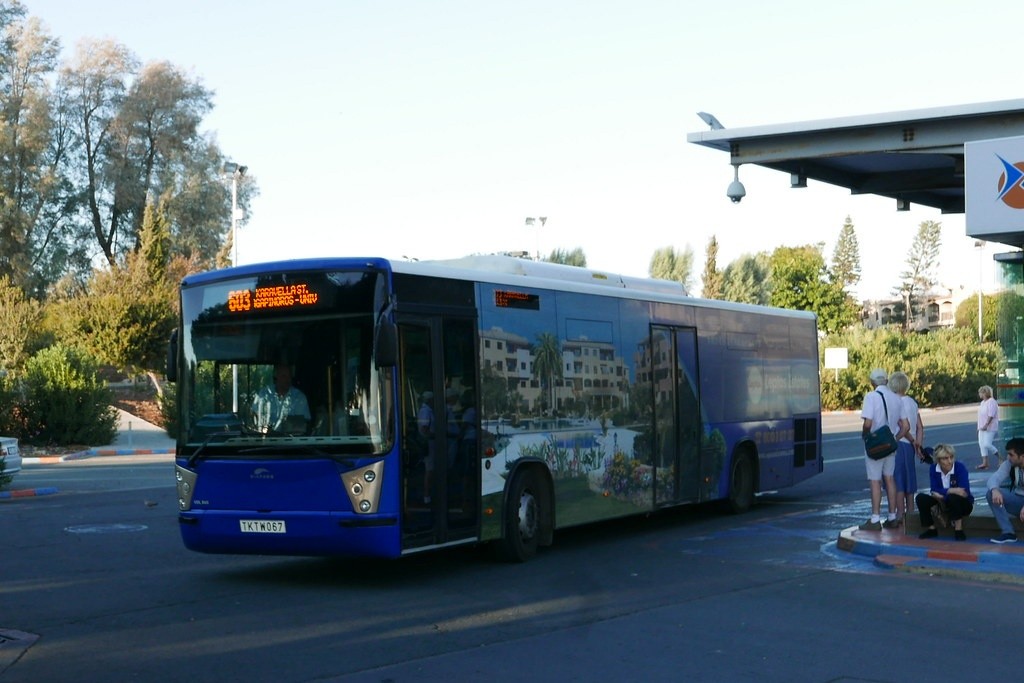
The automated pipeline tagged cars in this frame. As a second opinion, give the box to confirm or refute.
[0,435,22,482]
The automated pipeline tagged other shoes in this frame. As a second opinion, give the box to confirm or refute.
[896,514,903,522]
[883,518,898,527]
[976,464,989,469]
[996,457,1005,468]
[858,518,882,530]
[954,529,966,540]
[919,527,938,539]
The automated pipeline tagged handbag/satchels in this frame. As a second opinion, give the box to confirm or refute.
[865,425,898,461]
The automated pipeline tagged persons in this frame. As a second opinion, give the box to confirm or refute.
[886,372,924,524]
[986,437,1024,544]
[916,444,974,542]
[859,367,910,531]
[419,384,476,507]
[250,365,311,434]
[976,386,1004,470]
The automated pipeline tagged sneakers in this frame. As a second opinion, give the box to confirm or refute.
[990,532,1017,544]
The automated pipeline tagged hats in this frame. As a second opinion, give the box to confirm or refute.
[869,368,888,380]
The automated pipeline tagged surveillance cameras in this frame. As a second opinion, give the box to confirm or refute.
[727,182,746,204]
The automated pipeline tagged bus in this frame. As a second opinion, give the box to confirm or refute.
[167,254,824,565]
